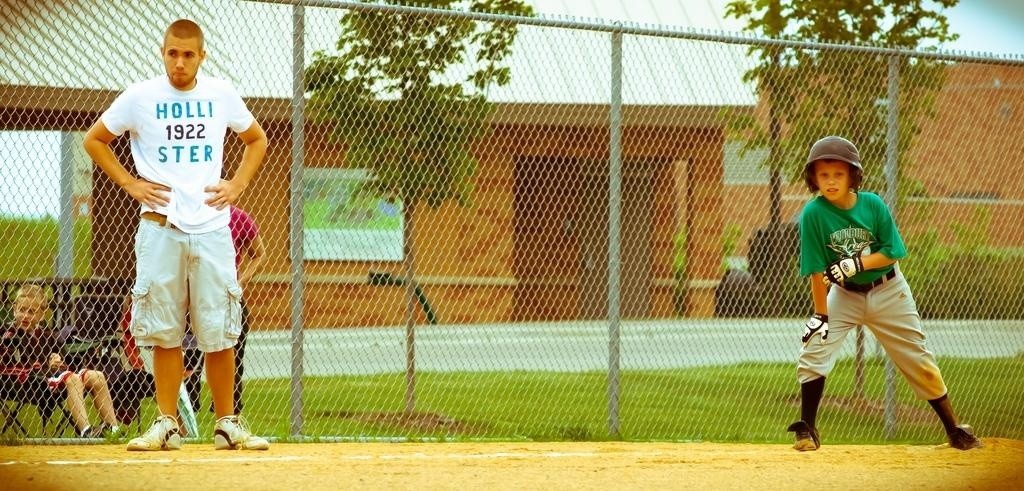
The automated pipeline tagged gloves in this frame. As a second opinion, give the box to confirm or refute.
[800,315,829,347]
[823,256,864,291]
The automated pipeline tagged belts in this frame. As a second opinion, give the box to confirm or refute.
[142,211,173,229]
[846,267,896,292]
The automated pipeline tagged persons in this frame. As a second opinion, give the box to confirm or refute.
[0,283,125,441]
[184,167,266,415]
[82,20,272,452]
[786,137,983,451]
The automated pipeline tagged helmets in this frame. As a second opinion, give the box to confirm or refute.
[801,137,864,193]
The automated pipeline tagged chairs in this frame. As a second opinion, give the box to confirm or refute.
[0,278,154,439]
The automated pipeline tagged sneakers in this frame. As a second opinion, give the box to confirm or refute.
[211,417,267,450]
[81,423,124,438]
[946,424,980,449]
[787,420,820,449]
[125,417,180,451]
